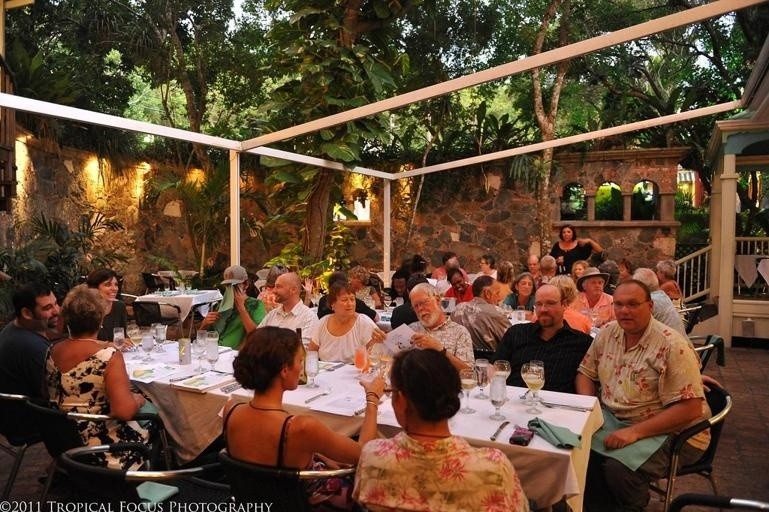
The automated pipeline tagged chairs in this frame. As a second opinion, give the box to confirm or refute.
[0,271,769,512]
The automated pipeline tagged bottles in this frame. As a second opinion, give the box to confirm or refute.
[295,327,306,385]
[558,263,566,274]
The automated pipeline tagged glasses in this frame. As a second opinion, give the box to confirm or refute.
[611,302,641,309]
[383,385,400,397]
[533,301,557,308]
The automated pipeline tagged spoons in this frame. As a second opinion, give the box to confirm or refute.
[305,383,336,404]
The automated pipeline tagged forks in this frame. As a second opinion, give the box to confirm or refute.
[539,400,586,413]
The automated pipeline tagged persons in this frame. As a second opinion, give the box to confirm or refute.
[1,224,724,512]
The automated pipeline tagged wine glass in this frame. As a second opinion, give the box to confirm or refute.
[383,296,392,314]
[165,279,192,297]
[590,307,598,331]
[113,323,166,362]
[192,329,219,372]
[353,344,384,380]
[459,359,546,420]
[306,351,319,388]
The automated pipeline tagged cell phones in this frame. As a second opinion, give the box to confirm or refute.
[510,428,534,446]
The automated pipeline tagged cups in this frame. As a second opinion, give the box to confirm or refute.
[178,338,191,365]
[516,305,525,322]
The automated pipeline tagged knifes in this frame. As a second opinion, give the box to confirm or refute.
[354,400,383,416]
[490,421,510,440]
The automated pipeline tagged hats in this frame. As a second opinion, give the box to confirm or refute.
[220,265,248,285]
[577,267,610,291]
